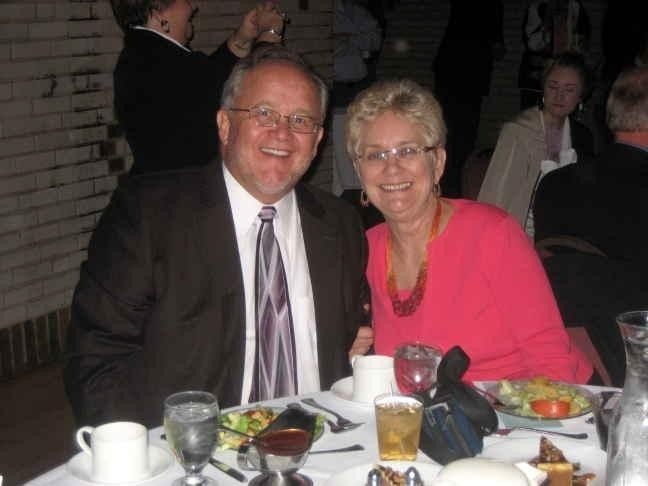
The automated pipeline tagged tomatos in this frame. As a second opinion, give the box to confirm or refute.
[531,399,571,420]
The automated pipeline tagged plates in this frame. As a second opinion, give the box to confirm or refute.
[480,373,598,422]
[212,395,324,453]
[327,455,438,485]
[481,432,608,485]
[331,373,401,406]
[64,443,172,485]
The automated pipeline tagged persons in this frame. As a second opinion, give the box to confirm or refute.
[531,63,648,389]
[432,1,508,196]
[601,2,646,143]
[62,44,372,445]
[110,0,290,173]
[519,2,595,162]
[342,73,596,397]
[476,51,595,235]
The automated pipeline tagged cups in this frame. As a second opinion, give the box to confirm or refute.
[372,391,422,459]
[75,422,148,484]
[393,342,441,402]
[346,353,393,402]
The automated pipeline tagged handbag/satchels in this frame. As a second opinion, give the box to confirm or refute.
[410,345,497,466]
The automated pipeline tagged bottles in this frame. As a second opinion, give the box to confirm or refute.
[609,310,647,485]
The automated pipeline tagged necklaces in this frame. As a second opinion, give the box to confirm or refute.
[380,201,450,315]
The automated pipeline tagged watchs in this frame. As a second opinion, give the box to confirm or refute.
[260,24,285,38]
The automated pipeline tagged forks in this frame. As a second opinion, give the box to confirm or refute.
[478,426,589,441]
[285,396,354,441]
[303,390,365,433]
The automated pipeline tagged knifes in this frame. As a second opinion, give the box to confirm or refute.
[157,432,245,486]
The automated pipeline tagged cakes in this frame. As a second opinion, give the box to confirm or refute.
[535,438,593,486]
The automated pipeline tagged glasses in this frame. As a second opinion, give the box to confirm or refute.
[358,145,436,165]
[229,105,323,133]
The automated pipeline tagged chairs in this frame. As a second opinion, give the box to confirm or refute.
[535,234,603,256]
[458,166,498,198]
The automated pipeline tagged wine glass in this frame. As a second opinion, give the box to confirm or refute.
[238,423,315,486]
[159,391,220,486]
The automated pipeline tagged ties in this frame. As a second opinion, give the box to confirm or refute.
[247,204,297,403]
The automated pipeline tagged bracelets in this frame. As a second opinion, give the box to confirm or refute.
[229,32,252,51]
